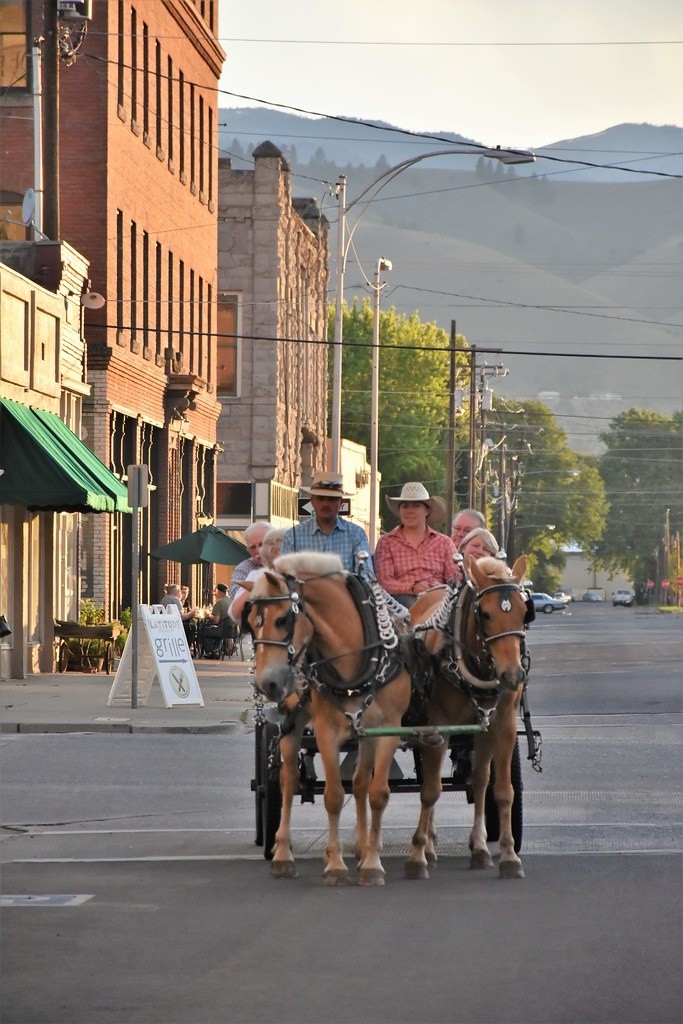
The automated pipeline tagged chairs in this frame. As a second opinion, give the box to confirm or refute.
[183,615,244,662]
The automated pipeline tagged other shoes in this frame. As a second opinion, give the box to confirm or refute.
[203,653,220,660]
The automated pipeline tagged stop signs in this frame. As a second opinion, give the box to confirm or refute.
[677,577,683,585]
[662,580,671,590]
[647,580,654,589]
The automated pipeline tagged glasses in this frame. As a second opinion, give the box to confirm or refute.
[311,480,344,494]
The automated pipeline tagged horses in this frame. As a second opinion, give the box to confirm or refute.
[227,553,529,885]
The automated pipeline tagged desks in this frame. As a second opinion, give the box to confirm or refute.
[193,617,210,658]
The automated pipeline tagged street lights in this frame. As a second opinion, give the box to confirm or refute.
[370,257,393,559]
[331,149,541,482]
[499,470,581,551]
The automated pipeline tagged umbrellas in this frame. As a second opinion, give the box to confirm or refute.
[148,525,252,606]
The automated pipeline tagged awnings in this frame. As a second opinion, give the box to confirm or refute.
[0,399,141,515]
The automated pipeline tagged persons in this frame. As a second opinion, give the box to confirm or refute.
[451,509,485,551]
[375,482,462,610]
[458,528,502,575]
[282,471,375,587]
[158,585,198,621]
[181,586,189,612]
[204,583,234,657]
[228,522,274,622]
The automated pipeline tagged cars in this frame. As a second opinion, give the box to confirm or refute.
[612,589,636,607]
[530,593,566,614]
[554,592,573,605]
[582,592,602,602]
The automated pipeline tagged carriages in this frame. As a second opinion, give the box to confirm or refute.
[230,555,548,890]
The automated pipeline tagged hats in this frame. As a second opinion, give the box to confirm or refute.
[385,481,447,523]
[300,470,356,497]
[217,584,228,592]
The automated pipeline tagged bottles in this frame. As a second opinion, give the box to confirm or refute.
[196,604,212,616]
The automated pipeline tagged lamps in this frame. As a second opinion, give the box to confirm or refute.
[68,290,106,310]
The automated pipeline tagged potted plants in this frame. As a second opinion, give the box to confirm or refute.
[74,598,132,672]
